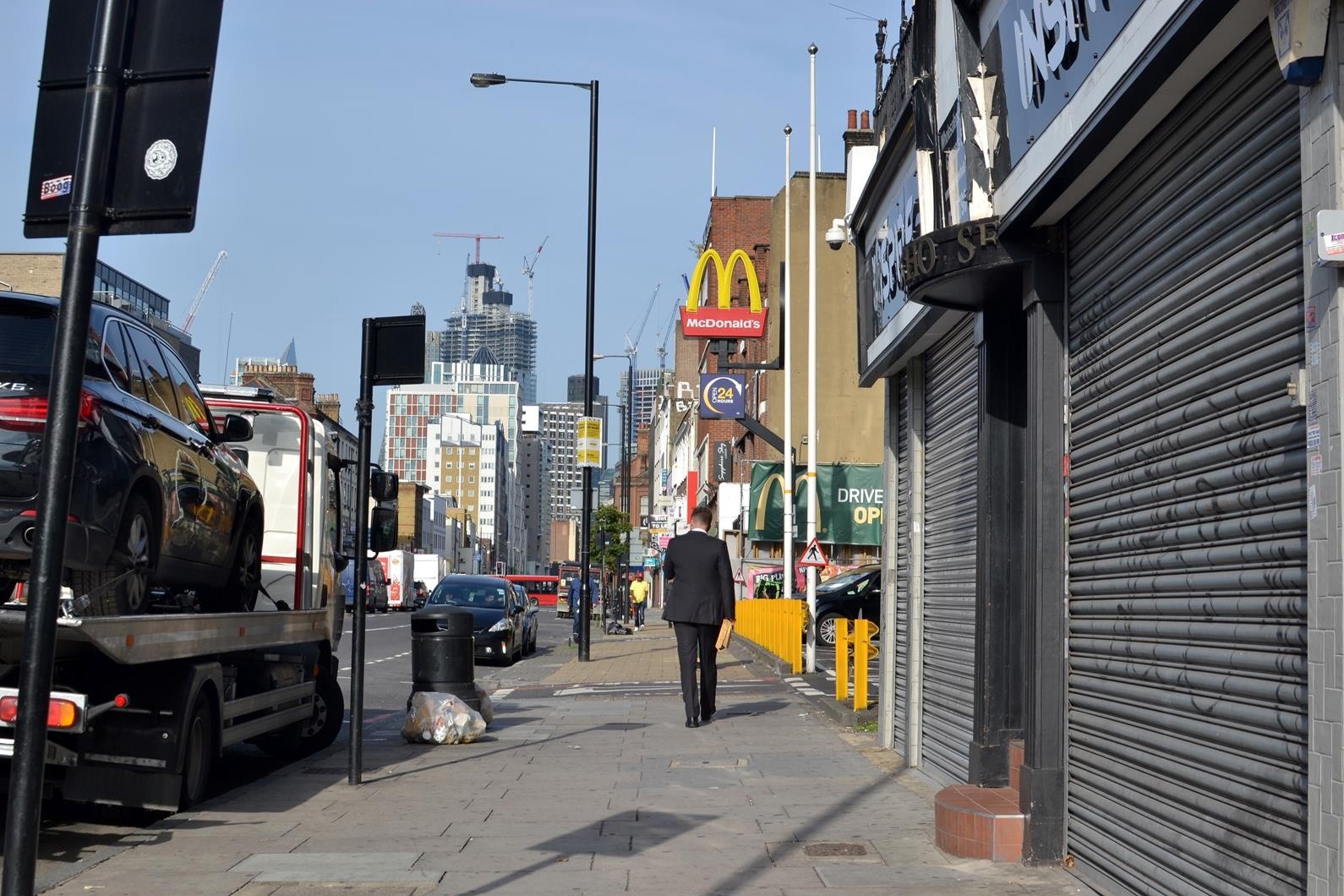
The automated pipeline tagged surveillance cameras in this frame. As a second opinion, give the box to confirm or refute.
[827,228,845,250]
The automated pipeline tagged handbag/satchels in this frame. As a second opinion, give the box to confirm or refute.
[400,690,487,745]
[474,682,492,726]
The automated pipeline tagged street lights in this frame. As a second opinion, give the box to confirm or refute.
[470,72,599,663]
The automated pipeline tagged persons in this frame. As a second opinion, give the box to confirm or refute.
[480,591,499,604]
[660,506,735,728]
[629,572,649,633]
[444,589,463,603]
[566,567,598,644]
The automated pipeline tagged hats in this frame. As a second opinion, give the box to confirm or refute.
[638,572,643,579]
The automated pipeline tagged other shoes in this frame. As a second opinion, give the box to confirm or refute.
[635,627,638,631]
[639,624,644,631]
[573,634,579,644]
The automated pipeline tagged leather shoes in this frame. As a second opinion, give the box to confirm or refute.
[685,717,699,728]
[701,706,716,721]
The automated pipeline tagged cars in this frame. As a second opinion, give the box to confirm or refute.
[413,581,432,610]
[426,574,541,663]
[783,563,881,647]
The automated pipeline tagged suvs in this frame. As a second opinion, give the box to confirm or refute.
[0,287,265,617]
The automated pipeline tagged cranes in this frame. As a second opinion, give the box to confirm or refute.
[434,233,501,263]
[623,283,681,455]
[181,250,227,333]
[520,235,548,320]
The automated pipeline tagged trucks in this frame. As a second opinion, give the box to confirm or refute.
[558,564,603,618]
[0,382,400,833]
[366,549,413,611]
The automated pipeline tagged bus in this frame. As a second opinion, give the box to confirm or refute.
[480,574,558,605]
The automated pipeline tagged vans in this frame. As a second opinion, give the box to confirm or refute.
[340,555,391,614]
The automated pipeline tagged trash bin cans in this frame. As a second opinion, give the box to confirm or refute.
[406,604,482,714]
